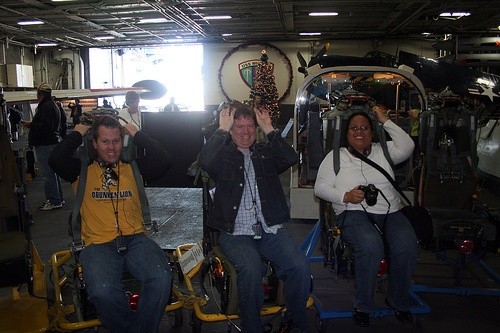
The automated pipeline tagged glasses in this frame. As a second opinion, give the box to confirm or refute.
[348,126,371,131]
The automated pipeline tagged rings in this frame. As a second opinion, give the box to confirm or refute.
[376,107,379,109]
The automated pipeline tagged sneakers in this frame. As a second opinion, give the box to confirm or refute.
[39,200,65,211]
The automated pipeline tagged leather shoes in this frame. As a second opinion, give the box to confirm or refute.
[384,295,413,324]
[352,306,370,328]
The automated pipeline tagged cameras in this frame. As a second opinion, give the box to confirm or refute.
[359,184,378,206]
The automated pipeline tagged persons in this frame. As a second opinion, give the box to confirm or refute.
[48,116,174,333]
[197,100,314,333]
[314,105,420,328]
[8,83,179,212]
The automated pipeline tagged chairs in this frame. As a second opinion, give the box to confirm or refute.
[201,116,295,314]
[73,130,168,314]
[331,101,421,279]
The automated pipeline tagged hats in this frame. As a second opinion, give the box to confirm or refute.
[37,82,52,93]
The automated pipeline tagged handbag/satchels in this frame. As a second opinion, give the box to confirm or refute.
[400,205,433,242]
